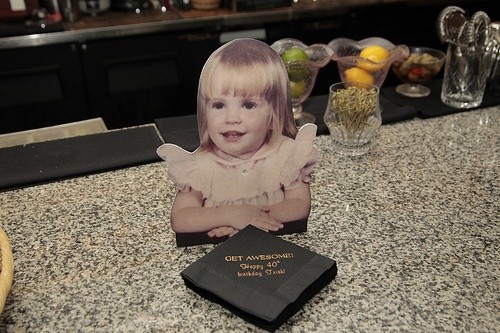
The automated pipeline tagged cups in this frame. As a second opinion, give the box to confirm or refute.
[323,81,382,147]
[441,40,496,110]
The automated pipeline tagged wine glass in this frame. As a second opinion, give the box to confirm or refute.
[392,47,445,97]
[326,37,409,115]
[267,38,336,129]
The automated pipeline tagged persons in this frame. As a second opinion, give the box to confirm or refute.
[156,37,320,238]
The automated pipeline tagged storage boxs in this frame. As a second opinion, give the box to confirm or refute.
[179,223,338,332]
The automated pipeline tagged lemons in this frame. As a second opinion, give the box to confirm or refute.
[357,45,390,72]
[344,67,374,90]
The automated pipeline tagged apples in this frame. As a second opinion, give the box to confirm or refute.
[281,48,311,82]
[289,80,306,98]
